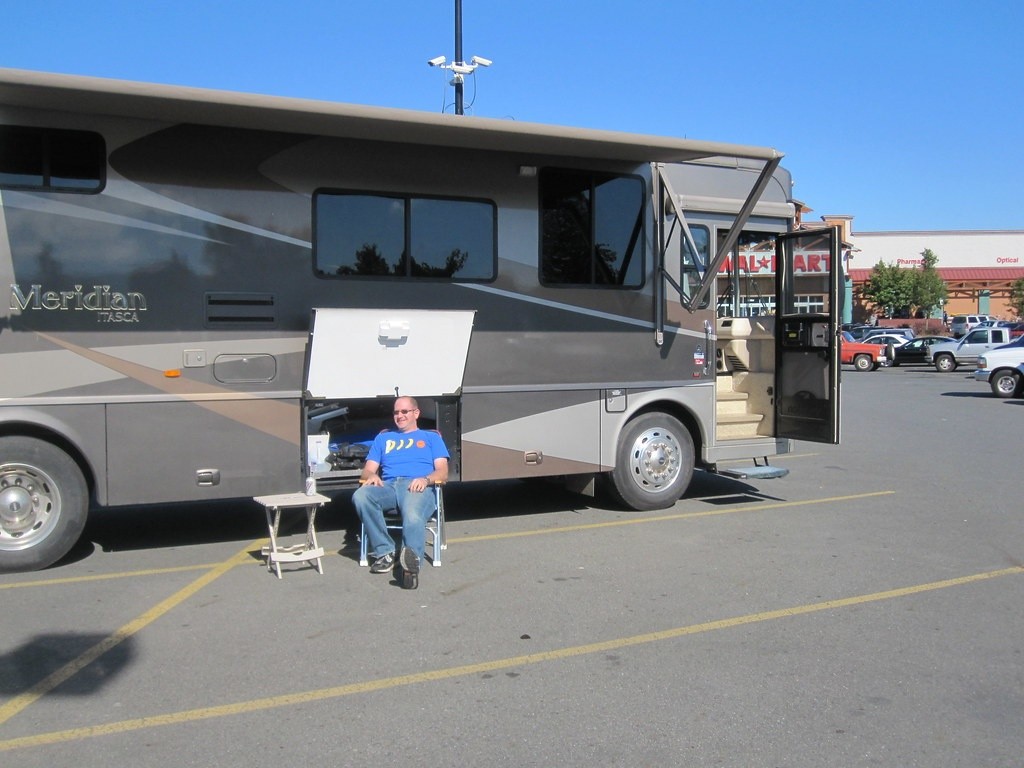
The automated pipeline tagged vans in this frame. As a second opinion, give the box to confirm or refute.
[860,328,916,343]
[851,324,894,340]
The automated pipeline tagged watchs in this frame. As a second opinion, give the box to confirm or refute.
[424,476,430,483]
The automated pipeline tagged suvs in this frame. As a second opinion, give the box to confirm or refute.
[842,331,887,373]
[974,334,1024,398]
[952,315,1000,339]
[928,327,1014,373]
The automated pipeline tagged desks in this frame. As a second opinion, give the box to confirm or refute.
[254,492,332,578]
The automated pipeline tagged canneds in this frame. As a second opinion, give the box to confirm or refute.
[305,477,317,496]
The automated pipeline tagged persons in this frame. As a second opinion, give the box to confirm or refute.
[352,397,451,590]
[943,311,948,326]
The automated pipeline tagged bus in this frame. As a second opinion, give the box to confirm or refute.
[0,71,846,577]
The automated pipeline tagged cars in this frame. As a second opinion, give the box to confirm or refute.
[861,334,910,351]
[893,337,960,367]
[969,320,1009,333]
[1009,323,1024,338]
[836,323,862,336]
[1002,322,1024,330]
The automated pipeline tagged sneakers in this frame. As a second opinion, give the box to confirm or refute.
[370,549,400,572]
[399,546,421,589]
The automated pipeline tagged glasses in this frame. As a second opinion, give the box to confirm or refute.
[393,409,417,415]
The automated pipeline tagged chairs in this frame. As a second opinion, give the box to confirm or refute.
[360,429,448,567]
